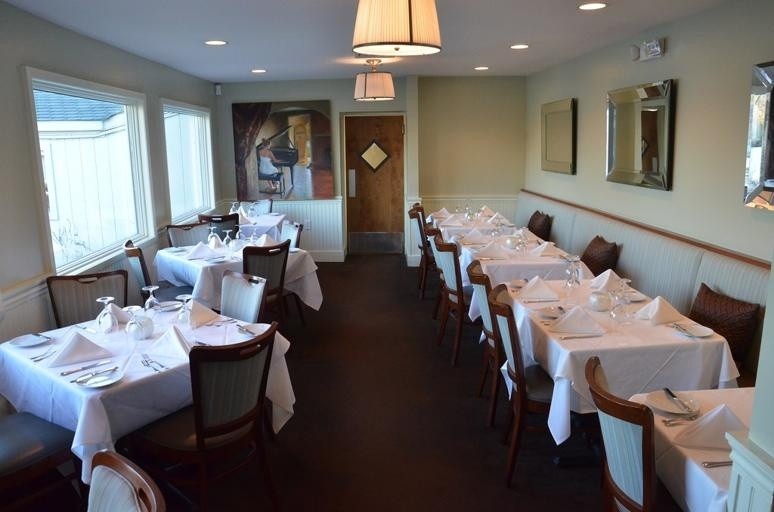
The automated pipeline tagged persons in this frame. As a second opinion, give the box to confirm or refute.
[260,138,282,191]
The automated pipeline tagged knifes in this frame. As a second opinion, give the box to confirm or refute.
[481,258,504,261]
[523,299,560,303]
[673,322,692,335]
[30,349,57,362]
[664,387,693,412]
[236,324,257,337]
[75,323,88,330]
[31,332,51,340]
[70,366,118,383]
[188,258,204,260]
[62,360,110,376]
[559,335,601,340]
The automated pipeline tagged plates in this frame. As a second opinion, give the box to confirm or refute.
[647,390,700,414]
[237,324,271,337]
[510,279,525,288]
[8,334,52,347]
[158,301,183,312]
[674,325,714,338]
[624,291,647,301]
[288,248,300,253]
[76,368,124,388]
[271,213,279,216]
[540,305,574,319]
[522,297,552,309]
[206,251,226,264]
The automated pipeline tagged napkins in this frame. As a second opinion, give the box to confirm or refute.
[635,295,686,326]
[186,242,206,258]
[256,235,275,246]
[549,307,606,336]
[189,303,219,329]
[96,306,131,323]
[593,271,625,291]
[49,331,117,367]
[516,276,556,301]
[206,248,227,262]
[172,325,215,357]
[676,405,754,449]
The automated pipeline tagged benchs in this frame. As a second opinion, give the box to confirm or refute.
[257,171,285,199]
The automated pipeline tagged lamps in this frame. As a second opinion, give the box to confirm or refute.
[353,59,395,102]
[352,1,442,57]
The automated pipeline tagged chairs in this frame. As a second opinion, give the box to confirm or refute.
[85,450,163,512]
[467,260,512,425]
[409,200,623,280]
[585,357,676,510]
[432,231,474,365]
[218,272,271,324]
[46,270,129,328]
[3,412,85,511]
[244,239,291,319]
[162,324,279,504]
[166,200,304,248]
[488,284,578,482]
[123,242,193,303]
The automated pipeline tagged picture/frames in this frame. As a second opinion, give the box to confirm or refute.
[540,98,575,174]
[743,61,774,213]
[605,78,672,192]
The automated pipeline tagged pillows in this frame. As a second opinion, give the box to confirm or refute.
[691,282,764,388]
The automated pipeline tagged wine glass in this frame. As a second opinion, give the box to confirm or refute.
[207,224,258,246]
[142,286,161,314]
[607,290,632,325]
[563,255,584,291]
[175,294,192,324]
[228,201,268,220]
[96,297,118,334]
[454,205,527,257]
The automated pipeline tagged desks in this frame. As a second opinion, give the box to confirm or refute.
[497,279,740,447]
[150,240,322,311]
[0,302,296,481]
[629,385,755,508]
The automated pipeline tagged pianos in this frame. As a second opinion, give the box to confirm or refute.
[256,125,298,186]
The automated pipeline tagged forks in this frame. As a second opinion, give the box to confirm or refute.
[141,353,164,368]
[215,321,237,327]
[205,319,234,326]
[141,360,159,372]
